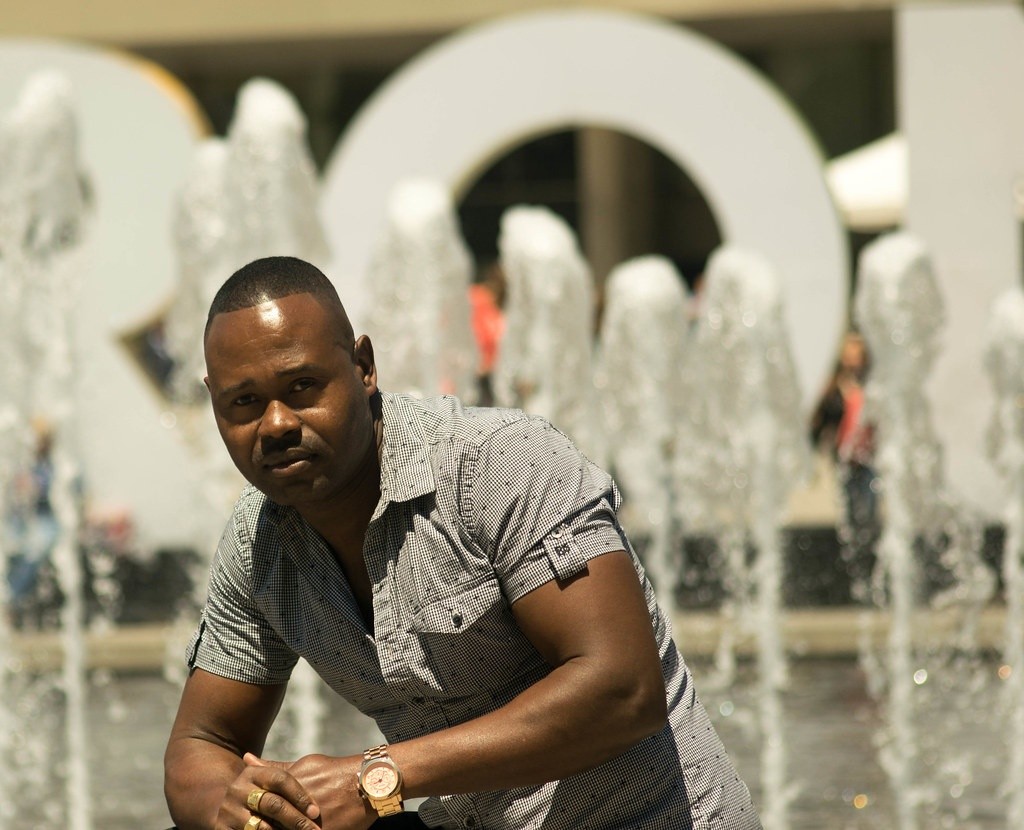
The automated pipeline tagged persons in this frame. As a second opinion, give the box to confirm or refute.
[808,326,878,606]
[163,257,765,830]
[468,244,509,407]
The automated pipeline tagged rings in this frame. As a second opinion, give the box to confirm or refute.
[244,815,263,830]
[246,789,267,813]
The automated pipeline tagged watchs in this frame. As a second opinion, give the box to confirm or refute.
[358,745,406,819]
